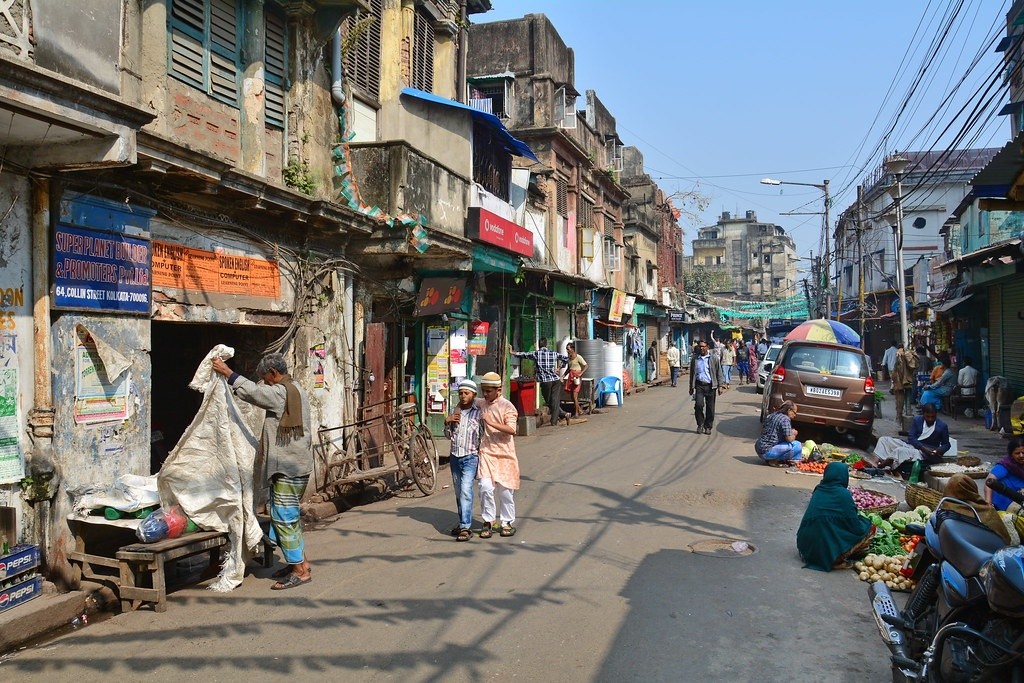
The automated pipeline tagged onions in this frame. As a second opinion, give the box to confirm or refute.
[847,485,893,509]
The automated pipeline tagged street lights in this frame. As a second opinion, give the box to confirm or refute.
[760,178,832,322]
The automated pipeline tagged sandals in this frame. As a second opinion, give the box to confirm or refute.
[457,528,474,541]
[451,527,461,536]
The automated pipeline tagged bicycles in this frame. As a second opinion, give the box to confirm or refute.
[388,402,443,495]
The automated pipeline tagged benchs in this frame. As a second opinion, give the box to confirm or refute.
[117,516,275,615]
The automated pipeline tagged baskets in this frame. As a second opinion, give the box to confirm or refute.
[905,483,944,512]
[853,486,899,519]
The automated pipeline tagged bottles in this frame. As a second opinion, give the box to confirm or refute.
[908,458,922,485]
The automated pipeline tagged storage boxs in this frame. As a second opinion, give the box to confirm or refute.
[923,472,987,500]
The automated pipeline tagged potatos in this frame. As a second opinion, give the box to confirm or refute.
[854,550,918,593]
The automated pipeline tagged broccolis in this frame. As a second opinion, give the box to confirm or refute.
[888,504,934,534]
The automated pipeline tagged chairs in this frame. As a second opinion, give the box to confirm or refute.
[915,375,934,410]
[597,376,622,409]
[950,384,979,419]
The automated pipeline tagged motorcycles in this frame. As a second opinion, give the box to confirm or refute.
[869,496,1024,683]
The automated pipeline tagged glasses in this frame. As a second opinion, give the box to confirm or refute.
[791,409,796,415]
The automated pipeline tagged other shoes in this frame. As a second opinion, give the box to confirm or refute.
[565,413,571,425]
[697,423,704,434]
[705,428,711,435]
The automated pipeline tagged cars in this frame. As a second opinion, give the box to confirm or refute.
[761,339,875,448]
[757,345,785,393]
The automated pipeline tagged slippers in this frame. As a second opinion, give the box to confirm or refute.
[271,572,312,590]
[480,528,492,538]
[272,564,312,580]
[500,527,516,537]
[833,561,853,569]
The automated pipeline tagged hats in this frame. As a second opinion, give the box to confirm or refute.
[481,372,502,388]
[458,379,477,393]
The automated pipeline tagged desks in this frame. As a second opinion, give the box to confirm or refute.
[64,513,146,594]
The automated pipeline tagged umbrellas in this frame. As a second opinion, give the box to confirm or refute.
[783,315,860,346]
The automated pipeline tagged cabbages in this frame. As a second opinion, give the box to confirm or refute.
[857,510,893,535]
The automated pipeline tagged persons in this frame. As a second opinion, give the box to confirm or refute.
[443,379,484,541]
[984,435,1024,510]
[873,402,951,472]
[509,336,572,427]
[938,473,1011,544]
[689,339,724,435]
[795,462,876,573]
[646,335,771,387]
[451,372,520,538]
[880,341,978,415]
[560,342,589,419]
[210,353,311,590]
[754,400,803,468]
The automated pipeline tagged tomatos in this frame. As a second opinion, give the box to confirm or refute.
[899,534,926,551]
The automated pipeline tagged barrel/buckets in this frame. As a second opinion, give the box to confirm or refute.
[983,405,1004,429]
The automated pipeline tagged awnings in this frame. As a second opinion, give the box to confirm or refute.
[932,236,1021,270]
[522,263,598,290]
[933,293,975,311]
[963,128,1024,197]
[401,87,544,166]
[466,70,515,87]
